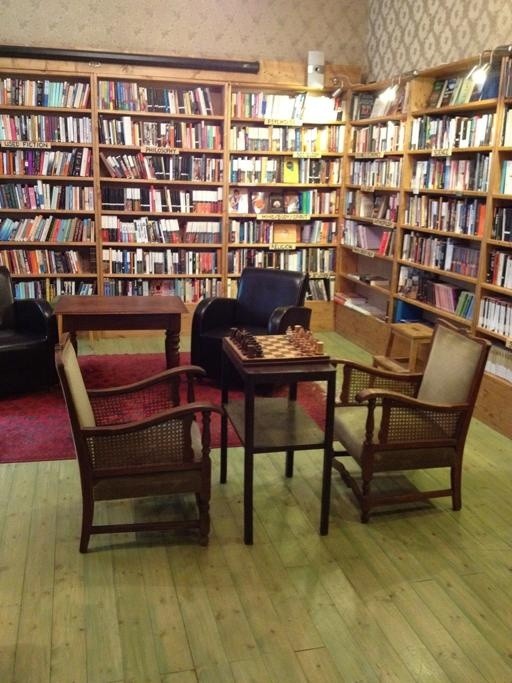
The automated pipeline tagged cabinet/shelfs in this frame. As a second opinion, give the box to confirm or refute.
[0,48,341,348]
[341,48,512,438]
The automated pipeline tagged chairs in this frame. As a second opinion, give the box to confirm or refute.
[330,319,492,524]
[54,333,224,554]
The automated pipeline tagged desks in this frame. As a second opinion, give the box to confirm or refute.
[220,343,335,544]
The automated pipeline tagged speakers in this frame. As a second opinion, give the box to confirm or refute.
[307,50,325,88]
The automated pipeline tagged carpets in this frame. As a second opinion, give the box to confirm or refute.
[0,351,327,463]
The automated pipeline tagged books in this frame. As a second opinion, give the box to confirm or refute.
[0,76,94,299]
[227,91,344,302]
[99,81,223,303]
[346,62,512,383]
